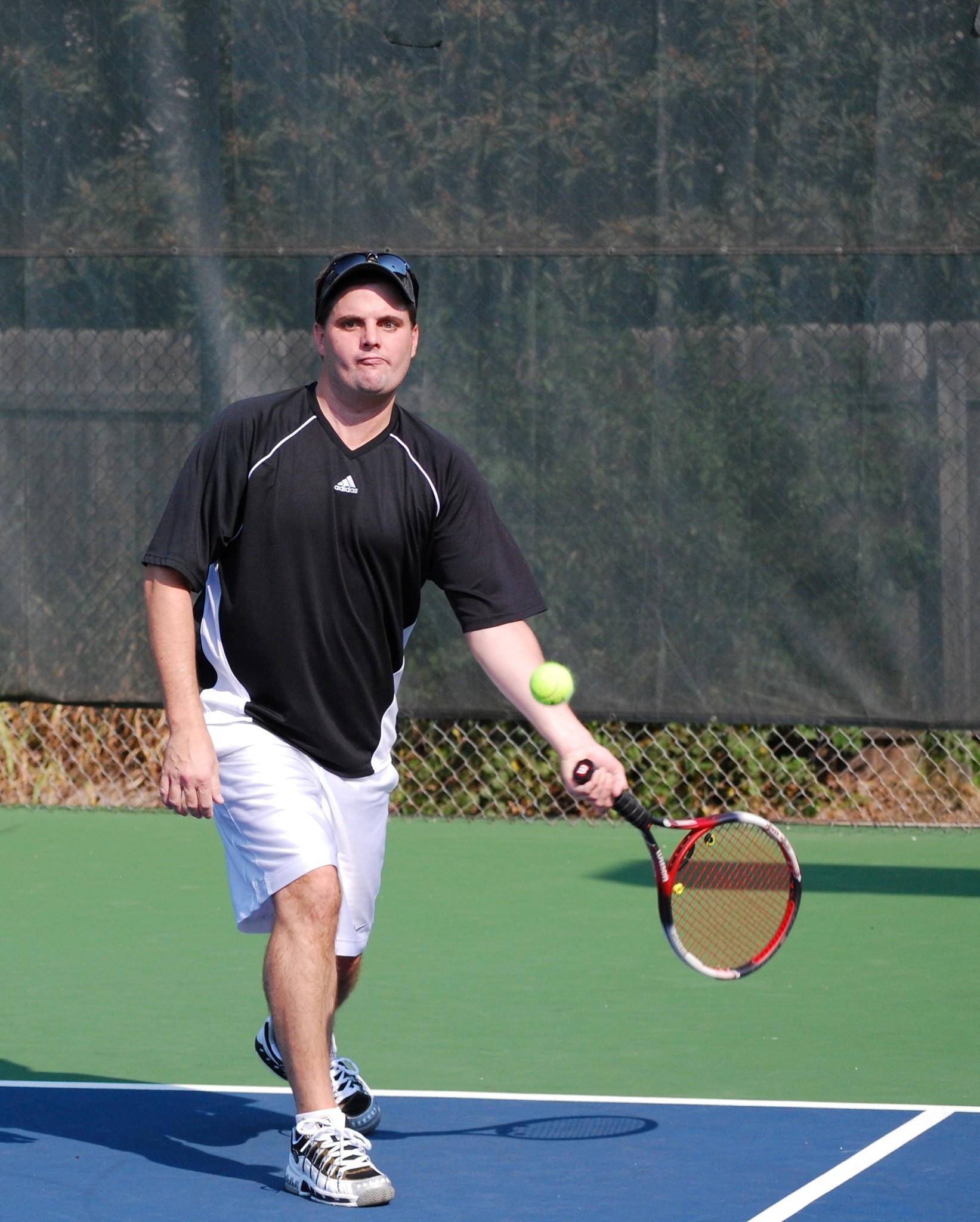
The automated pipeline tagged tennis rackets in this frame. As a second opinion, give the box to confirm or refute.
[573,759,801,981]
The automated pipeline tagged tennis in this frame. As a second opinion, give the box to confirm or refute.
[529,662,573,705]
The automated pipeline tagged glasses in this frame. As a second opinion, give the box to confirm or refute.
[316,251,418,311]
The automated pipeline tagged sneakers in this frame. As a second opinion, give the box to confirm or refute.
[253,1016,381,1134]
[282,1113,395,1207]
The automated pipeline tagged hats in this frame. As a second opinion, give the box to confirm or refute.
[315,255,417,306]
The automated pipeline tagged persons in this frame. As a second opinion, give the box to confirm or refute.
[142,253,629,1207]
